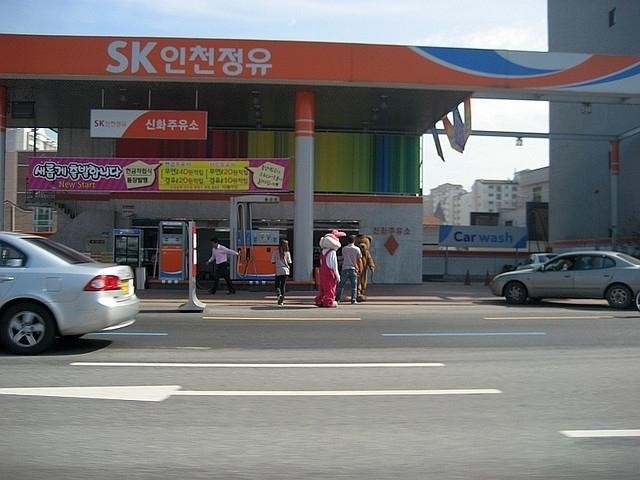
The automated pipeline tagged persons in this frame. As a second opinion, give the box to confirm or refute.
[205,238,241,295]
[270,239,293,306]
[336,235,363,304]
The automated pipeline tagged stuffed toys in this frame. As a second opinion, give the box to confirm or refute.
[315,228,347,307]
[354,234,376,302]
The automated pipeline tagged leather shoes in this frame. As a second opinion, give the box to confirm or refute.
[208,289,236,295]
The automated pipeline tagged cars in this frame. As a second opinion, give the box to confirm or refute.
[0,231,140,355]
[489,250,640,310]
